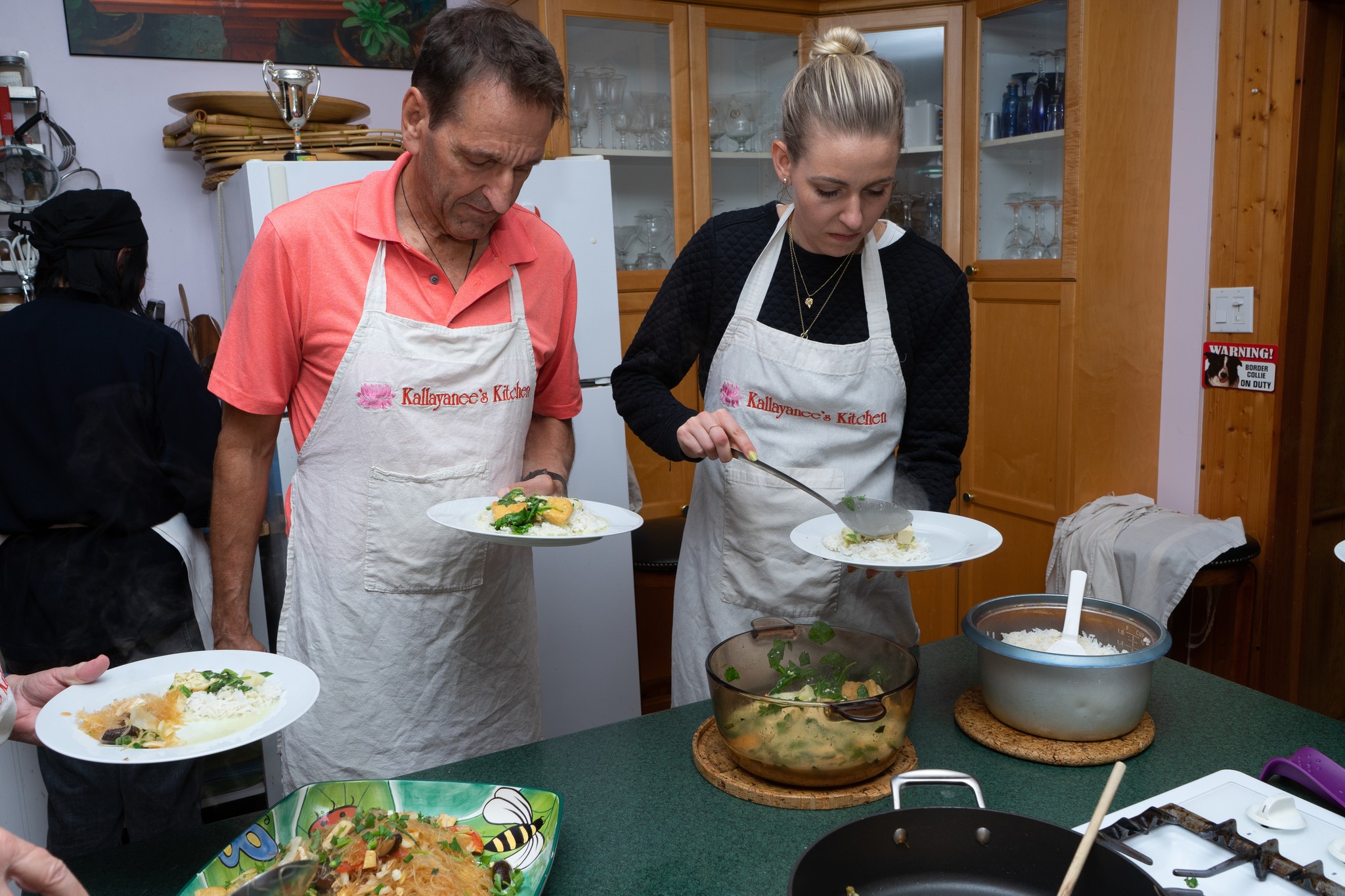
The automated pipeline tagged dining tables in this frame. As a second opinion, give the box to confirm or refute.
[33,637,1345,896]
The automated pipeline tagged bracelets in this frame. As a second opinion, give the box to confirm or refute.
[521,469,568,498]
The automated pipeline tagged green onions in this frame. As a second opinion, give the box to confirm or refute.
[223,806,414,895]
[115,731,160,748]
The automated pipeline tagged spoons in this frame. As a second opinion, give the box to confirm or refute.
[230,859,317,896]
[175,779,564,896]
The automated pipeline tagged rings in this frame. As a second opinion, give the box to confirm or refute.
[708,424,722,434]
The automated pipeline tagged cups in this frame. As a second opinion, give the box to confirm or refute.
[1046,94,1063,132]
[1019,94,1035,134]
[982,112,998,141]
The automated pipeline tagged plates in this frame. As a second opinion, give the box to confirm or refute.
[35,649,320,764]
[789,510,1004,571]
[425,496,643,546]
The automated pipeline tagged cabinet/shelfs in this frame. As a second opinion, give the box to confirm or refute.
[0,86,54,314]
[498,0,1178,715]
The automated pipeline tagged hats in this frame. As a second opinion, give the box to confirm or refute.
[30,188,149,255]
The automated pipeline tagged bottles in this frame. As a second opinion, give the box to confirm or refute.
[1001,84,1019,137]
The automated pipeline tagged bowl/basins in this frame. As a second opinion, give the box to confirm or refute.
[704,615,919,788]
[961,594,1173,742]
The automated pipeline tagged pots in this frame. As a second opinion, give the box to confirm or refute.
[786,768,1168,896]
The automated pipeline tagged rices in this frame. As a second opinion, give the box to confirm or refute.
[179,685,283,720]
[823,533,928,563]
[986,628,1150,655]
[474,496,609,537]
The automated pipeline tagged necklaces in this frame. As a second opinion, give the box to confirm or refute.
[789,212,858,340]
[402,170,478,294]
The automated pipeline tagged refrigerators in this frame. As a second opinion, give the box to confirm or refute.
[206,154,641,811]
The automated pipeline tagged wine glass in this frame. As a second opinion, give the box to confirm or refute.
[613,92,660,150]
[1003,193,1061,258]
[708,93,733,151]
[1011,49,1065,136]
[614,226,641,270]
[885,192,942,247]
[725,92,772,151]
[566,63,629,150]
[761,94,785,151]
[633,209,672,269]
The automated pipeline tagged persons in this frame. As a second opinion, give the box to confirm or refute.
[0,189,254,896]
[612,27,970,708]
[206,6,584,810]
[0,654,110,896]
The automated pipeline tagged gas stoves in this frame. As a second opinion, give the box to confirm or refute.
[1070,770,1345,896]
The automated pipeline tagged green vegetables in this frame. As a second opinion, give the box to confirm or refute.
[485,487,579,535]
[842,496,915,549]
[412,812,524,896]
[170,668,274,697]
[722,620,893,768]
